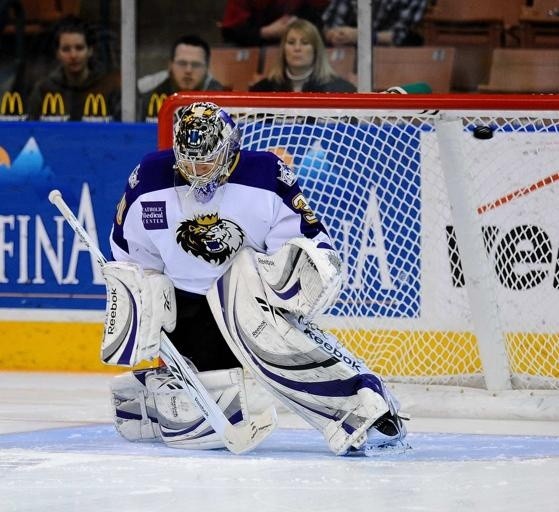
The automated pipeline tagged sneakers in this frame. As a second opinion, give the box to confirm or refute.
[365,397,407,448]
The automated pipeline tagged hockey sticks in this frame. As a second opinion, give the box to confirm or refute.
[48,189,278,455]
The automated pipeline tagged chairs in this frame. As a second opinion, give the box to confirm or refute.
[206,1,558,92]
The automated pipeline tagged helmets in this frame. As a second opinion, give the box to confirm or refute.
[173,101,240,190]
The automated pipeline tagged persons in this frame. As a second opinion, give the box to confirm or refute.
[138,35,229,125]
[96,100,413,461]
[218,1,328,47]
[27,21,120,123]
[320,1,428,50]
[246,17,358,95]
